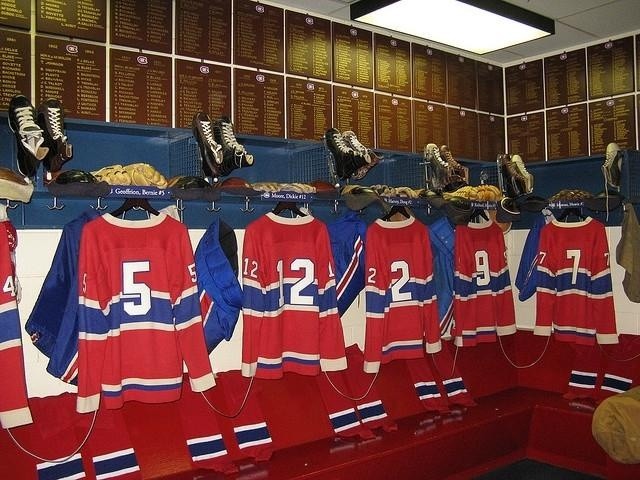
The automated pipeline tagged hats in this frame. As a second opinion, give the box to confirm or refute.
[213,178,337,199]
[1,163,213,204]
[341,183,622,212]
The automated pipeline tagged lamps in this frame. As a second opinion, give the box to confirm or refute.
[350,0,555,56]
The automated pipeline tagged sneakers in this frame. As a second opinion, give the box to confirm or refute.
[497,153,534,197]
[424,144,468,193]
[8,94,73,176]
[192,112,255,178]
[601,143,623,188]
[326,128,384,181]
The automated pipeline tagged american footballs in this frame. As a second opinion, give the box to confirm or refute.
[221,177,254,190]
[44,170,65,197]
[311,181,336,192]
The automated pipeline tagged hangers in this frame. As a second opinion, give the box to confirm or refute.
[382,205,410,221]
[557,208,586,222]
[469,209,489,222]
[110,195,162,217]
[273,200,308,217]
